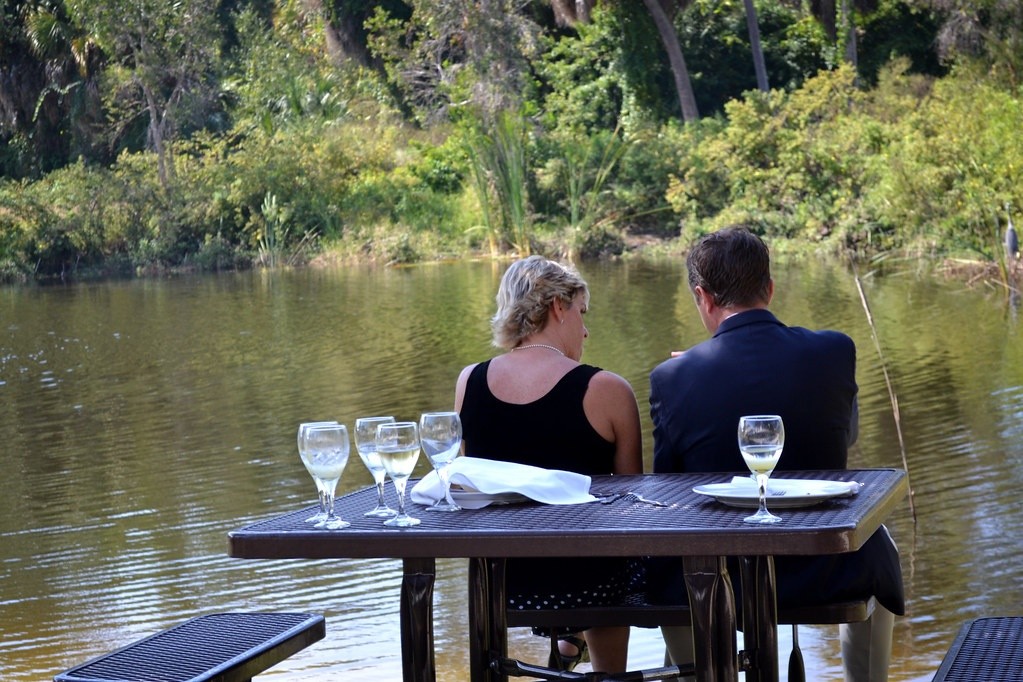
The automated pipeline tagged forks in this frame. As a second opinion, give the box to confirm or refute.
[624,493,668,507]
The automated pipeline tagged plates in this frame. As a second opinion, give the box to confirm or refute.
[693,478,853,509]
[449,491,527,506]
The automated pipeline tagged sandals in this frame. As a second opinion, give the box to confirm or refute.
[547,634,590,672]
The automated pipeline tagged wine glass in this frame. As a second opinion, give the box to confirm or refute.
[297,421,341,523]
[354,416,396,517]
[375,422,421,527]
[419,412,462,512]
[305,425,350,529]
[738,415,785,524]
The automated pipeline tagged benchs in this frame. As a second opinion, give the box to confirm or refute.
[503,593,876,682]
[52,612,326,682]
[931,615,1023,682]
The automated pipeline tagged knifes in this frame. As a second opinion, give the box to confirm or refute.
[600,490,627,504]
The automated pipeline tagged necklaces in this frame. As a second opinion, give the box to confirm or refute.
[511,344,565,355]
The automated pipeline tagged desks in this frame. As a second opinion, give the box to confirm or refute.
[233,468,910,682]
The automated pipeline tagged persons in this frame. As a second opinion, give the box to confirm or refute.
[647,225,906,682]
[453,256,646,674]
